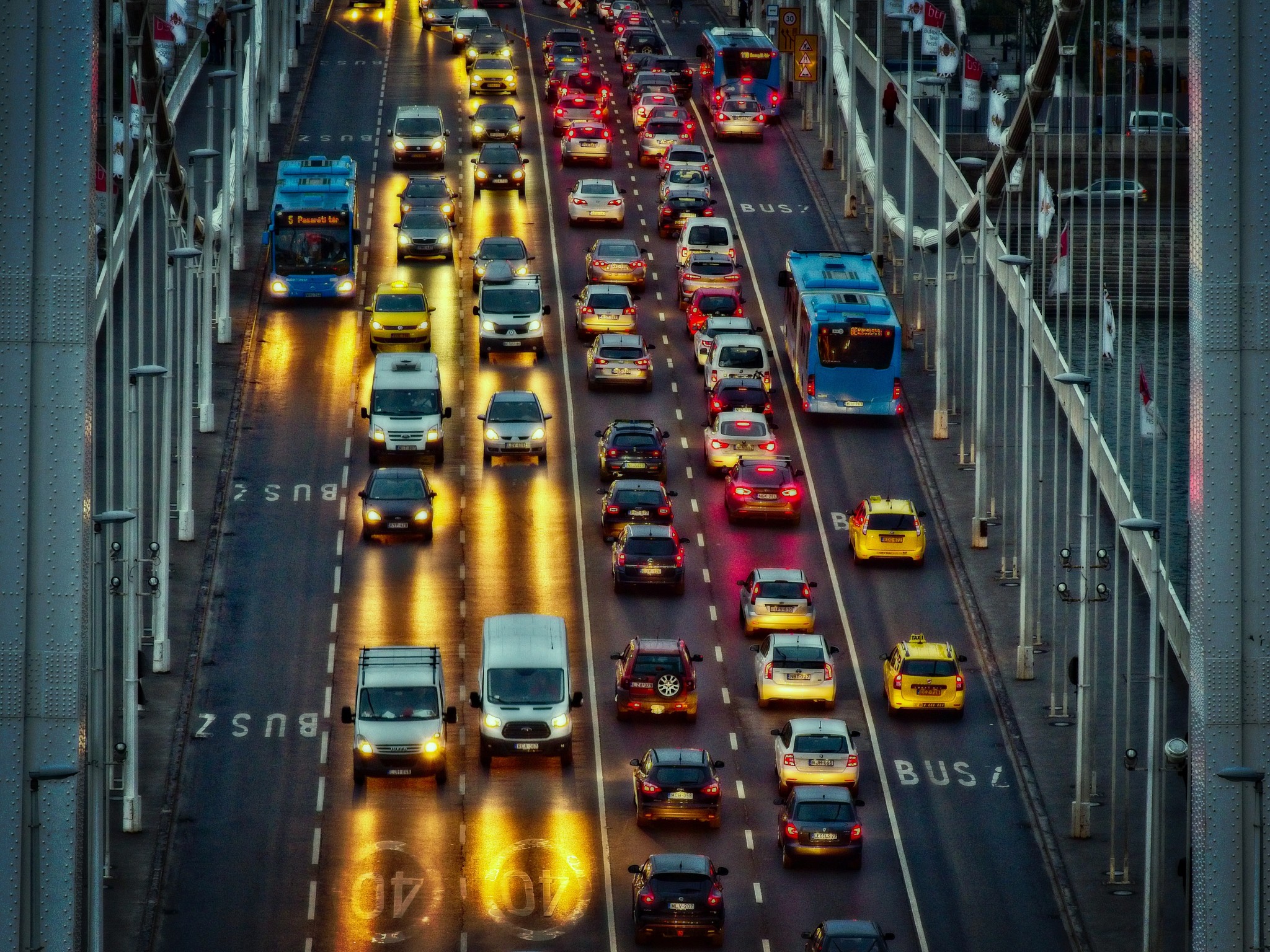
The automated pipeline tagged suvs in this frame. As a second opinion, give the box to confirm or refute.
[341,645,458,788]
[359,351,456,468]
[848,496,926,565]
[611,524,692,595]
[610,637,704,720]
[879,633,968,720]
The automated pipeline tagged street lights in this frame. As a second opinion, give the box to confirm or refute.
[118,3,261,833]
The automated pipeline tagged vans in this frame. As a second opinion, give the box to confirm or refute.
[469,613,584,773]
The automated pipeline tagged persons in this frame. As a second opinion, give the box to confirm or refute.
[739,0,747,28]
[328,243,346,259]
[816,56,838,95]
[280,232,322,264]
[883,82,899,128]
[533,671,559,696]
[990,57,1000,90]
[670,0,683,25]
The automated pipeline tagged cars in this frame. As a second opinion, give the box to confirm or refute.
[750,633,841,710]
[630,747,726,830]
[836,5,1189,948]
[722,455,805,525]
[699,410,780,474]
[351,0,802,391]
[774,785,866,871]
[594,418,670,484]
[477,389,553,463]
[627,851,729,950]
[596,480,677,541]
[771,718,861,802]
[800,920,895,952]
[737,568,818,638]
[359,467,436,543]
[705,377,776,429]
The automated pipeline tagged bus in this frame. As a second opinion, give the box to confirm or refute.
[777,247,904,419]
[261,155,361,302]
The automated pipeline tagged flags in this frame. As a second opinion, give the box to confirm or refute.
[1100,283,1116,363]
[1137,368,1168,440]
[881,0,1071,300]
[94,0,187,261]
